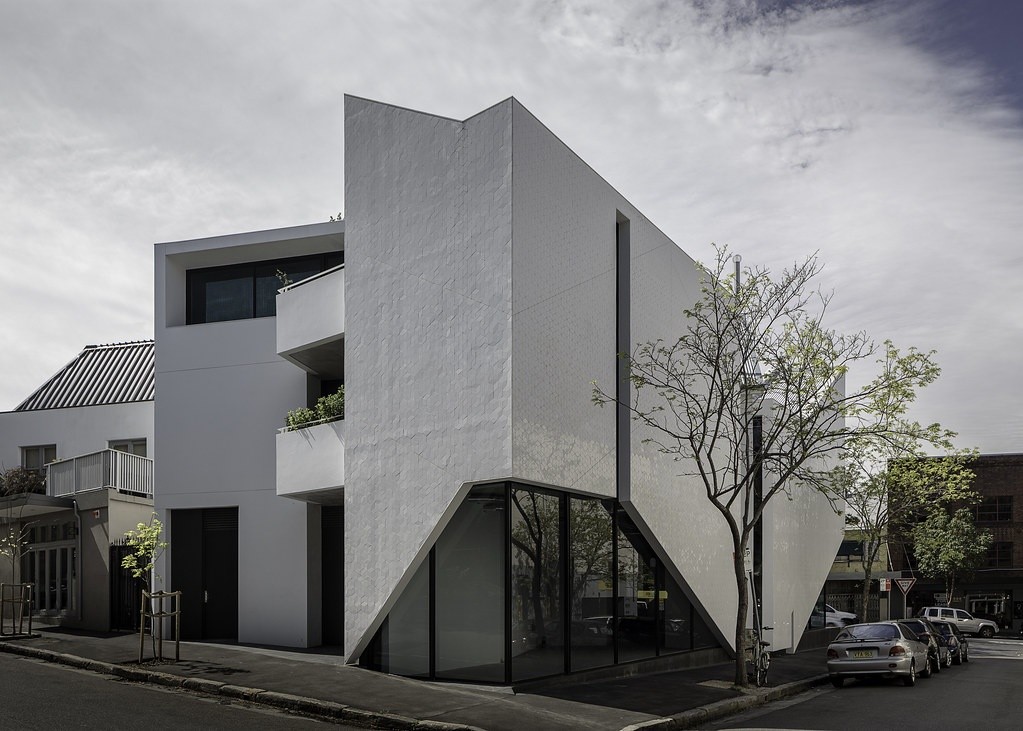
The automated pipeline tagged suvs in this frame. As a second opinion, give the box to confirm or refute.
[913,606,1000,638]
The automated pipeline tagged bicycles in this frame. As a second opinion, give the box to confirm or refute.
[744,625,775,687]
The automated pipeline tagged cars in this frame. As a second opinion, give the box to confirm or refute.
[882,618,952,671]
[809,608,846,628]
[929,620,969,665]
[825,603,858,624]
[616,616,659,646]
[579,615,613,646]
[825,622,932,687]
